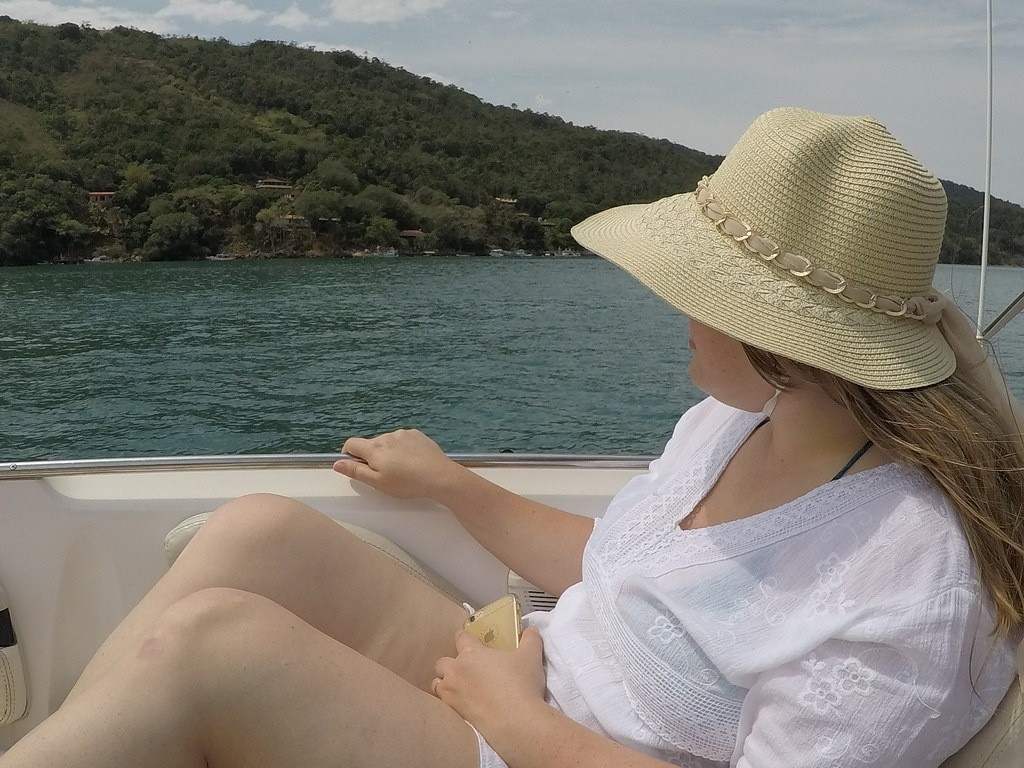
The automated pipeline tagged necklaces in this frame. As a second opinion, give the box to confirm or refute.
[830,437,876,482]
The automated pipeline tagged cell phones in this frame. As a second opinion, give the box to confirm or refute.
[462,592,524,650]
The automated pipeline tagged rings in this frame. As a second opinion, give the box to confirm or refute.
[434,681,441,695]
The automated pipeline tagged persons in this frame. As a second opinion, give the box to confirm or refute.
[0,106,1024,768]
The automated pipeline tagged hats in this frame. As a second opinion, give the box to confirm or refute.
[570,107,957,389]
